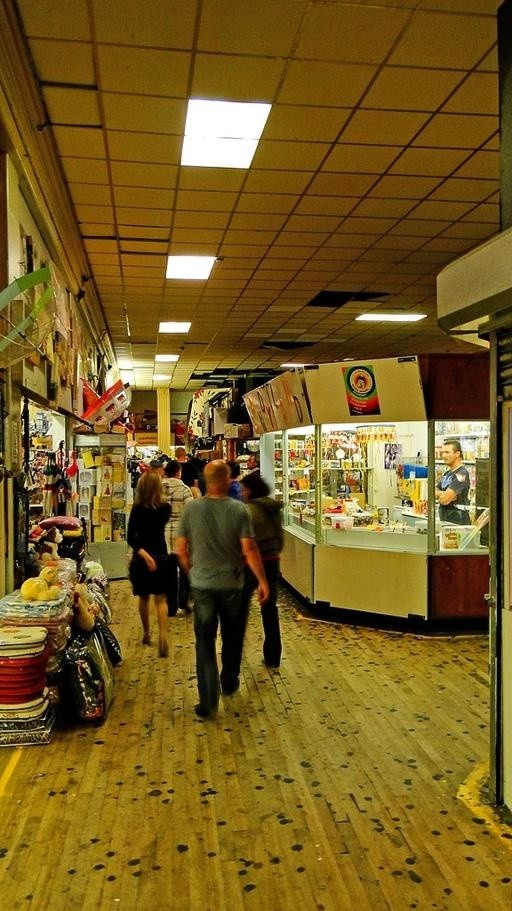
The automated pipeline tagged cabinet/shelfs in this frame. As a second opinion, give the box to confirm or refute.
[433,433,491,465]
[275,446,376,496]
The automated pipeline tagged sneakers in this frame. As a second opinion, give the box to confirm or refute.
[142,632,170,658]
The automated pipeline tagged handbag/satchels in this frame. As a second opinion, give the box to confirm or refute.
[94,616,123,666]
[64,623,115,729]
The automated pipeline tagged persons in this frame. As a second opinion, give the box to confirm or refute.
[124,471,172,659]
[138,446,249,619]
[233,473,286,669]
[176,459,274,717]
[435,440,472,526]
